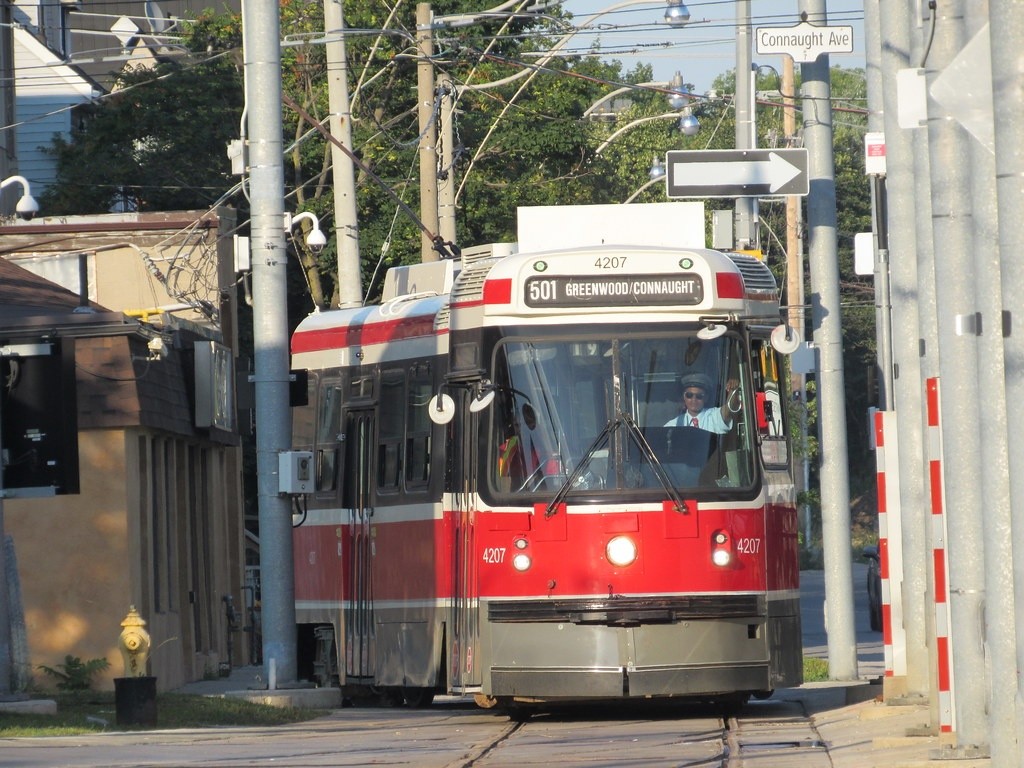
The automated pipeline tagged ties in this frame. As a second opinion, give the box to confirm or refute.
[692,418,699,428]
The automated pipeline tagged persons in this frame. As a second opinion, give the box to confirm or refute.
[499,412,560,478]
[663,373,739,434]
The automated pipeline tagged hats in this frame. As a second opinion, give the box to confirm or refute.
[681,373,713,393]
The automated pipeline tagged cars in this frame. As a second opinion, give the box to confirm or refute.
[861,538,882,632]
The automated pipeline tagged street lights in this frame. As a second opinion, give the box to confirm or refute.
[579,70,690,119]
[594,106,700,154]
[437,0,691,263]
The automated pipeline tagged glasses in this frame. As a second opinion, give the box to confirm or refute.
[684,392,706,399]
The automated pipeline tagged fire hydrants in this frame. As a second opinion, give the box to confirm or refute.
[117,607,153,677]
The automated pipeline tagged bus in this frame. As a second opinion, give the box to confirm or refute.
[290,199,803,721]
